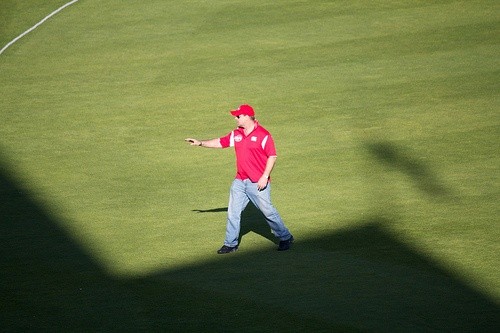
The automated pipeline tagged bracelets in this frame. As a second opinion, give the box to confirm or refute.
[199,142,203,146]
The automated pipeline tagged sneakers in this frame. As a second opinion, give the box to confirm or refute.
[217,245,239,254]
[277,236,295,251]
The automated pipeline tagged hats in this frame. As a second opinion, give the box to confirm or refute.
[231,104,254,116]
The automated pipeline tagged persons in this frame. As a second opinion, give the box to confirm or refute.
[185,104,295,254]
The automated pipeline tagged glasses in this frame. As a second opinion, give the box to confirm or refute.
[237,115,244,119]
